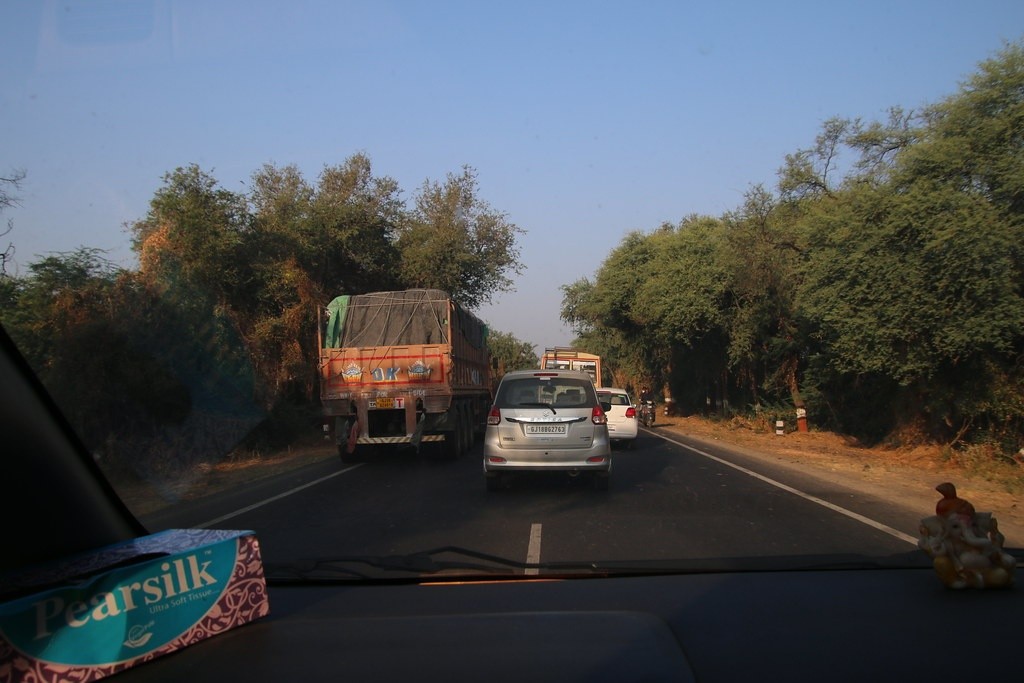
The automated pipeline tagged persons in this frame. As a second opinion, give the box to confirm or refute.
[638,387,658,422]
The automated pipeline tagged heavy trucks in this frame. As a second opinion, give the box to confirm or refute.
[316,288,499,463]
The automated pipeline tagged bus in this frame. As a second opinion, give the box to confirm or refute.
[538,346,601,409]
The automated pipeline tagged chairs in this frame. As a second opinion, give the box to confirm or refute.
[556,388,582,403]
[612,396,627,403]
[518,389,535,403]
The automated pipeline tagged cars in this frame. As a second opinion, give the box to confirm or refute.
[483,368,614,487]
[578,387,640,450]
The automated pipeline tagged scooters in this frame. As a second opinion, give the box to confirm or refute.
[640,400,657,427]
